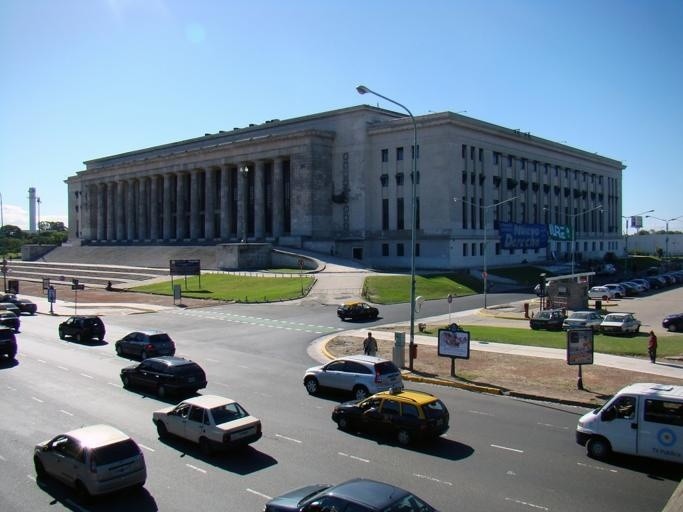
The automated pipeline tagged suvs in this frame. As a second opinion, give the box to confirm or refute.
[302,354,403,402]
[57,315,104,343]
[33,423,147,496]
[331,381,449,441]
[120,355,206,398]
[336,301,379,322]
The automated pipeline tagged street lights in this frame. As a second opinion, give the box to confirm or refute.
[645,214,683,273]
[452,194,522,308]
[598,209,654,281]
[355,85,416,372]
[542,205,602,281]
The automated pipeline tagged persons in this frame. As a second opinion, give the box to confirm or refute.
[539,276,549,298]
[364,333,378,357]
[648,331,657,364]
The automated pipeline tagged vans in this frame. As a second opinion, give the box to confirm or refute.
[115,329,175,361]
[575,382,682,463]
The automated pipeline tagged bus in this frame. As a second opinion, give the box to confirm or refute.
[534,271,596,296]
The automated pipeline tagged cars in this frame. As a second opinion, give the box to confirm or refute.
[562,311,603,333]
[0,292,36,362]
[529,309,567,330]
[600,313,641,335]
[262,477,439,512]
[152,393,262,452]
[662,312,683,331]
[587,270,682,300]
